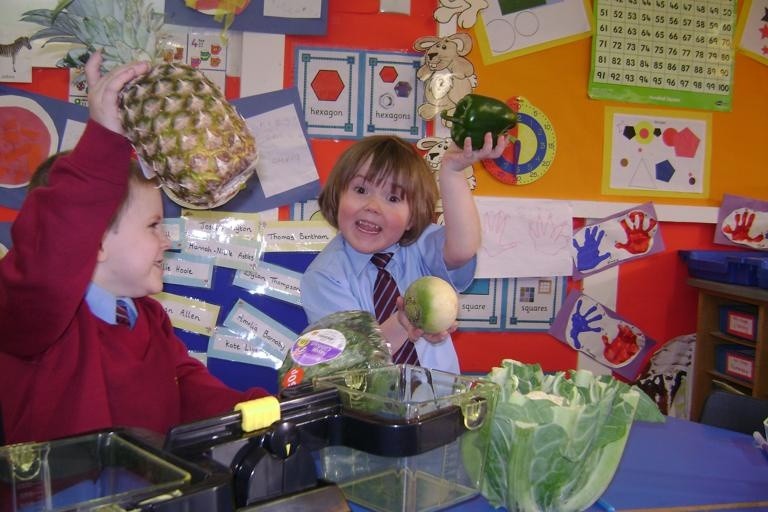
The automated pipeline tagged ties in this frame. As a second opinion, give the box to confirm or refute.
[116,305,130,328]
[370,252,422,398]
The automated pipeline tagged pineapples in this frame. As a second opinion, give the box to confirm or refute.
[19,0,258,208]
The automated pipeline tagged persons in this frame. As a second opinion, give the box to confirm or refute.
[300,131,509,374]
[0,49,278,512]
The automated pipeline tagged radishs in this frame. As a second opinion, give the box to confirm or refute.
[404,275,458,334]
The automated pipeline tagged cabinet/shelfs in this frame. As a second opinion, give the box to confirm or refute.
[685,278,768,424]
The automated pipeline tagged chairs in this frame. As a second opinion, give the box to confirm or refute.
[699,391,768,439]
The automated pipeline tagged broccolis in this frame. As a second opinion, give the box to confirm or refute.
[460,359,640,511]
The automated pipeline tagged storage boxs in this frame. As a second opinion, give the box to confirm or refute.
[741,256,768,289]
[678,250,756,285]
[719,305,757,341]
[716,345,755,382]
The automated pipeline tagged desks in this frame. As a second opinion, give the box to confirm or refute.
[23,416,768,512]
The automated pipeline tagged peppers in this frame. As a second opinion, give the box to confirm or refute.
[440,95,516,150]
